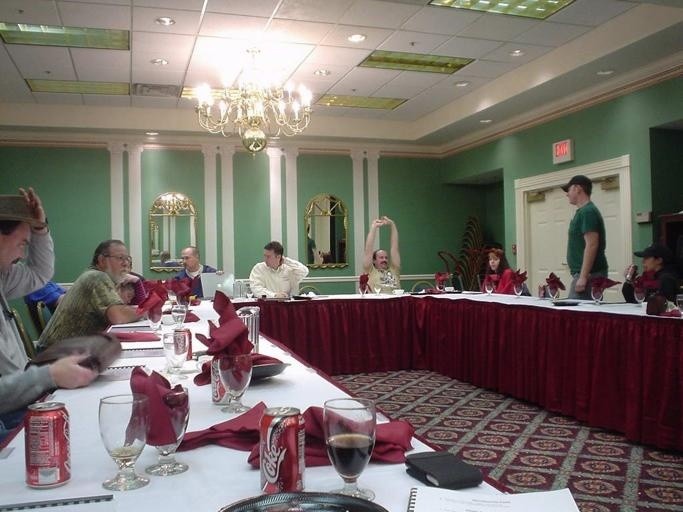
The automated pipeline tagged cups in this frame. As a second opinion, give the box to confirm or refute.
[435,284,444,291]
[391,289,404,296]
[233,304,260,355]
[676,294,683,312]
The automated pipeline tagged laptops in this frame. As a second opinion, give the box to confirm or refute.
[198,272,235,299]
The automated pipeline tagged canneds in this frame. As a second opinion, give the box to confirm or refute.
[260,405,306,494]
[173,327,192,361]
[625,263,637,283]
[25,401,72,489]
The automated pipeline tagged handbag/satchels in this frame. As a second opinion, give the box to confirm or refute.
[27,332,122,373]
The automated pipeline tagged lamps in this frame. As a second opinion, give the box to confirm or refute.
[195,42,314,155]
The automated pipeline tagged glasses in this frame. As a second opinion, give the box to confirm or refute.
[105,254,132,263]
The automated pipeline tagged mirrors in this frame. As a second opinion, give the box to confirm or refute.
[147,192,197,272]
[303,192,350,269]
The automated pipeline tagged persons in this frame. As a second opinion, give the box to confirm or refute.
[481,248,514,294]
[174,247,225,297]
[561,175,607,300]
[364,215,401,293]
[159,251,181,267]
[249,242,309,299]
[622,243,676,307]
[24,280,70,337]
[36,240,147,354]
[307,224,321,264]
[115,274,146,305]
[0,187,98,428]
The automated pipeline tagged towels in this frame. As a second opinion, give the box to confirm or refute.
[424,271,621,298]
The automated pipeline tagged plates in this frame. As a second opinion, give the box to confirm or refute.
[552,301,581,306]
[213,493,387,511]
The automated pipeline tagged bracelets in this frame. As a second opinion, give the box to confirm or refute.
[34,218,50,231]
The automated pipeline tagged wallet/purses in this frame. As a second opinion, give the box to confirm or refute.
[403,451,482,490]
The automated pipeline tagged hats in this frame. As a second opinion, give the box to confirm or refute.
[0,193,47,229]
[634,247,664,257]
[560,174,591,192]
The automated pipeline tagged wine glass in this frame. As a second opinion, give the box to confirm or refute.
[634,288,646,306]
[590,287,604,305]
[146,304,186,336]
[214,353,254,414]
[374,287,382,296]
[93,392,152,491]
[161,331,190,381]
[245,290,253,300]
[546,286,560,301]
[484,282,494,296]
[513,283,524,298]
[319,395,380,502]
[144,388,194,479]
[358,283,368,297]
